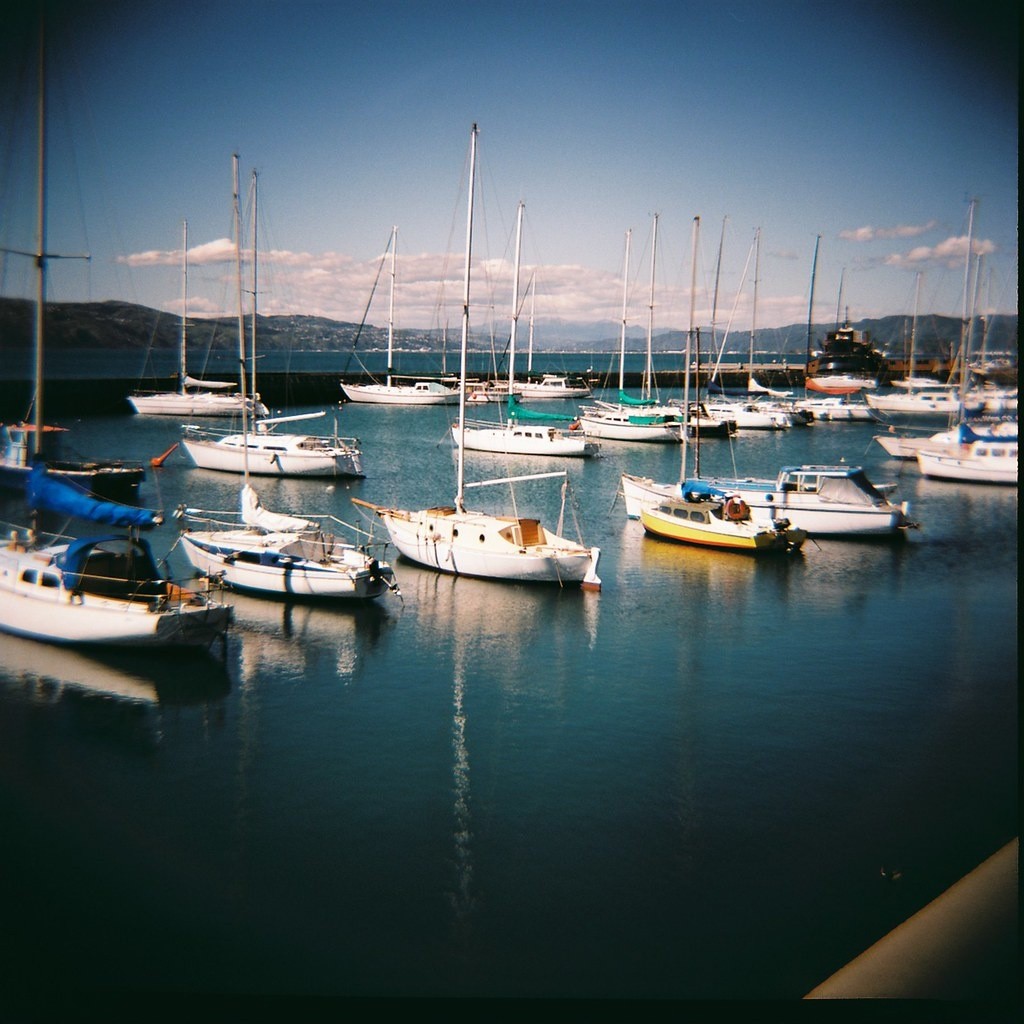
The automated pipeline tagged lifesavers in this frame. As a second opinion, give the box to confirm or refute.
[726,498,746,519]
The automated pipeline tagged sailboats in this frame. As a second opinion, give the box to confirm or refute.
[577,214,1017,441]
[447,204,601,457]
[181,169,366,478]
[640,327,807,554]
[802,266,889,394]
[340,213,460,404]
[349,123,602,592]
[872,196,1017,459]
[468,303,522,403]
[621,217,910,535]
[916,253,1018,484]
[127,218,270,415]
[0,0,146,505]
[0,39,236,645]
[176,153,402,599]
[514,269,592,397]
